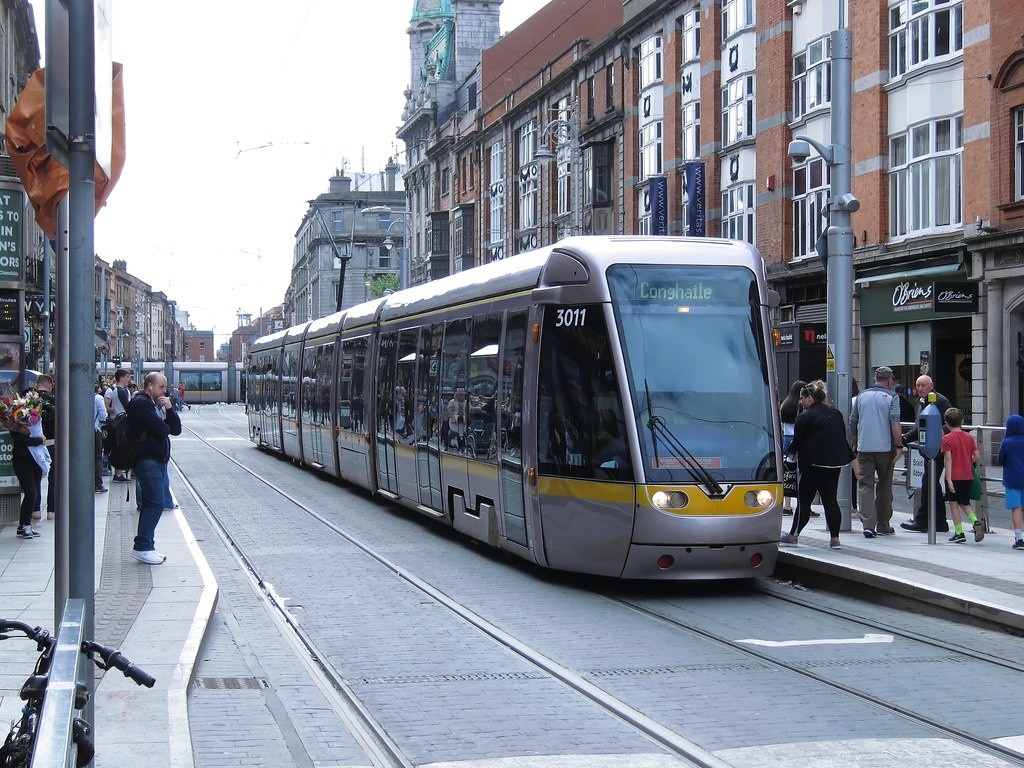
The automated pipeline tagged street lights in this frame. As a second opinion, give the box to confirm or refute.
[357,205,414,292]
[383,218,413,283]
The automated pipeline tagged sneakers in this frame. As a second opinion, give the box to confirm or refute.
[112,474,132,482]
[948,532,966,543]
[130,549,167,564]
[17,525,40,538]
[973,521,984,542]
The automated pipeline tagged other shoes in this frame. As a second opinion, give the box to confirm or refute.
[863,527,895,538]
[131,474,136,478]
[779,534,798,547]
[1012,539,1024,549]
[189,406,192,410]
[783,509,793,515]
[95,485,108,492]
[900,519,948,533]
[810,511,820,517]
[165,505,179,510]
[829,541,840,548]
[32,511,55,519]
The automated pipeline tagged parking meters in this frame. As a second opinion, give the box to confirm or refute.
[916,393,940,547]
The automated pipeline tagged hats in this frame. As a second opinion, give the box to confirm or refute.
[128,384,137,386]
[875,366,896,378]
[894,384,904,397]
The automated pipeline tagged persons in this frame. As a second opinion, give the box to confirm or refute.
[780,381,820,518]
[376,383,523,463]
[901,376,952,531]
[30,374,56,519]
[851,376,861,519]
[248,381,331,428]
[10,388,46,538]
[940,407,984,543]
[351,395,366,435]
[126,371,181,564]
[93,367,191,513]
[848,367,903,538]
[996,415,1024,550]
[778,379,863,549]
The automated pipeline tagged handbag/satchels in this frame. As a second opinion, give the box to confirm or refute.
[112,438,143,470]
[783,444,798,498]
[971,468,982,500]
[102,412,129,456]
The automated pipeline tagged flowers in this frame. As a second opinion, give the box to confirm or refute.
[0,384,43,433]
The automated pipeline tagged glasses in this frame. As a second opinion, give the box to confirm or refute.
[103,385,108,386]
[129,386,137,388]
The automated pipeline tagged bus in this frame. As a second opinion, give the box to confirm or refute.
[246,200,786,584]
[46,359,244,404]
